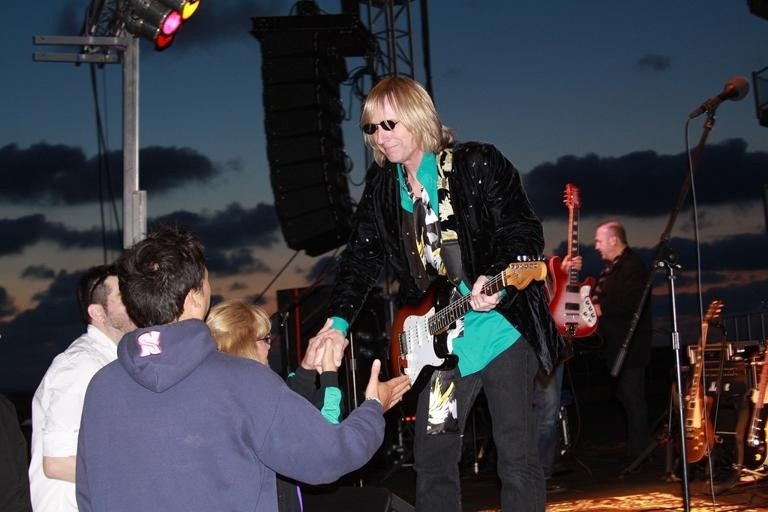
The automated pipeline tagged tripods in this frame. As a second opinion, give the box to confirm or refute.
[618,431,683,485]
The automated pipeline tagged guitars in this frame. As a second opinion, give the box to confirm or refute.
[681,300,724,462]
[734,338,768,476]
[390,254,548,390]
[548,183,600,341]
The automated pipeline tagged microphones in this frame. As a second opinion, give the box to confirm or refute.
[688,76,750,119]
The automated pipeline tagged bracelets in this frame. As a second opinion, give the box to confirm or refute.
[366,397,382,404]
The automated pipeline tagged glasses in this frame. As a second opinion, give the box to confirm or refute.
[364,121,399,134]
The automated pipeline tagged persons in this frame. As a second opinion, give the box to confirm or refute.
[525,249,586,495]
[305,71,581,511]
[202,299,350,510]
[583,219,655,477]
[74,220,414,511]
[26,262,145,512]
